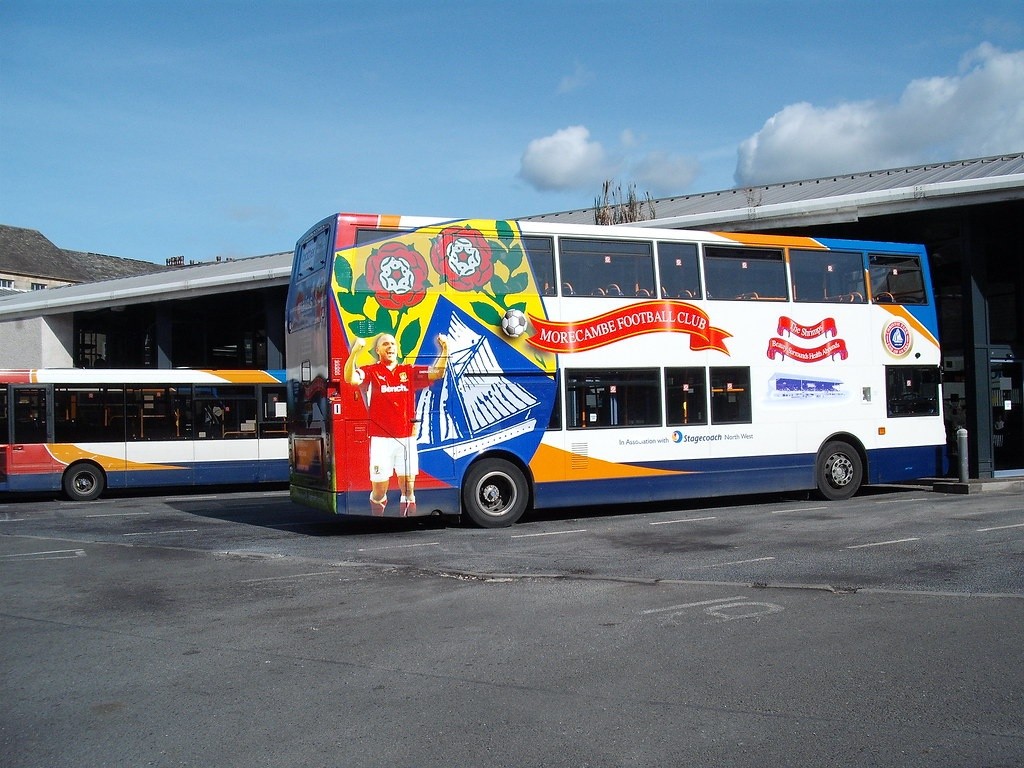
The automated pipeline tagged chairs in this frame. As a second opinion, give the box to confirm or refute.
[544,282,896,302]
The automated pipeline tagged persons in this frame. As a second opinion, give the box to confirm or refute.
[293,292,308,330]
[343,333,450,518]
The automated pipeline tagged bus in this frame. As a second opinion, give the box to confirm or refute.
[282,212,951,525]
[2,366,294,503]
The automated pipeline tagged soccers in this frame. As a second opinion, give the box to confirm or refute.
[501,309,530,338]
